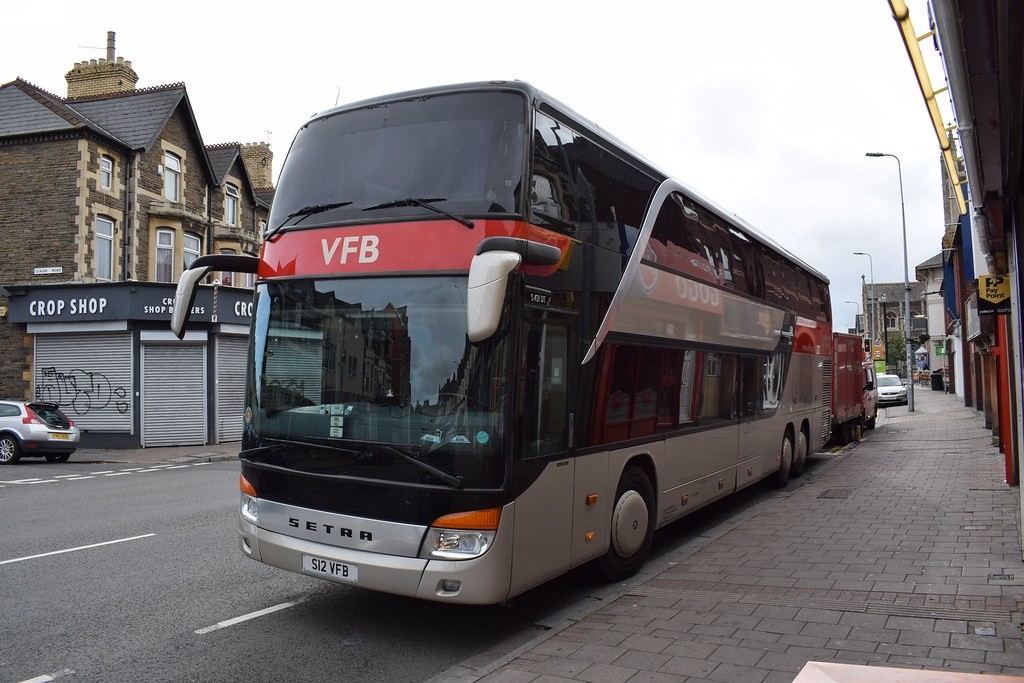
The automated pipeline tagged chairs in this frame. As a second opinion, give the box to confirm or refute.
[912,373,929,387]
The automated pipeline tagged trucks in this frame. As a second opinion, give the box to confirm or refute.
[834,332,878,446]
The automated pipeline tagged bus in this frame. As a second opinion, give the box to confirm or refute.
[170,79,833,604]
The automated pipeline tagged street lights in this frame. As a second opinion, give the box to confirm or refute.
[866,152,914,411]
[854,253,874,341]
[845,302,860,335]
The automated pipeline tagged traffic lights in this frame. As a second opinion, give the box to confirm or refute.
[864,338,870,352]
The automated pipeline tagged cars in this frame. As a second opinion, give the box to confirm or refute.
[876,375,908,407]
[0,398,80,465]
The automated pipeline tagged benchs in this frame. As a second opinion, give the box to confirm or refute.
[10,410,46,419]
